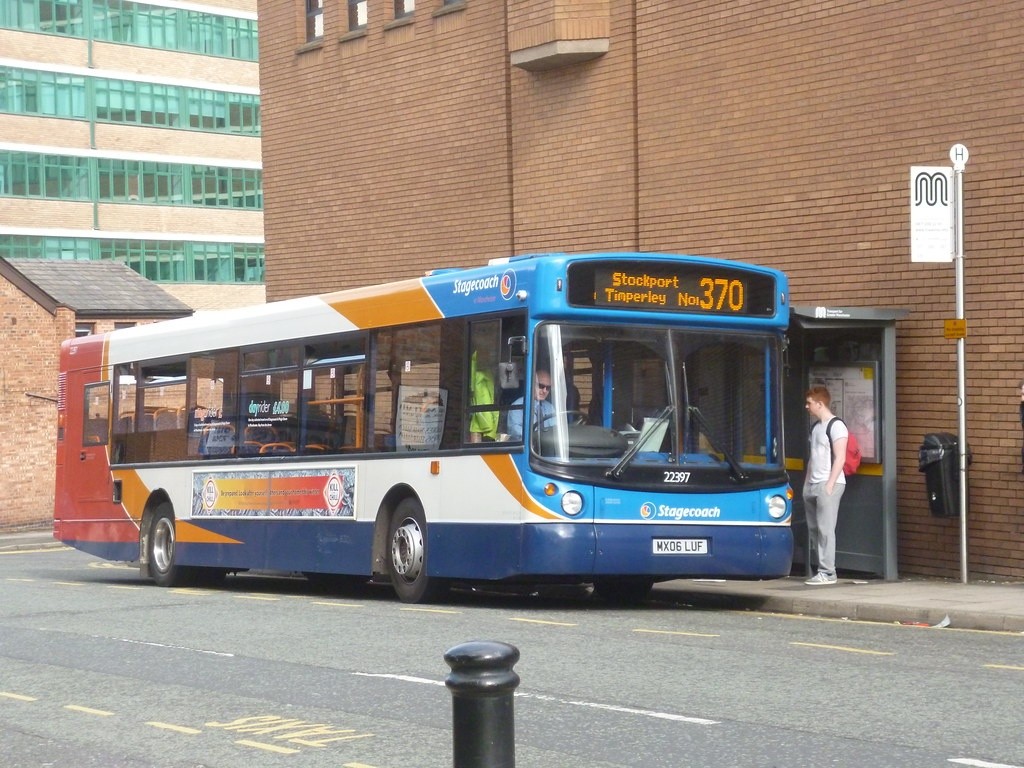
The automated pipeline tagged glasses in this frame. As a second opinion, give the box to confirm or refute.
[538,383,552,392]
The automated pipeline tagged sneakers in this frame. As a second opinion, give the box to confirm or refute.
[804,573,838,585]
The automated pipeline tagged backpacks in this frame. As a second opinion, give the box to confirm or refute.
[809,416,862,477]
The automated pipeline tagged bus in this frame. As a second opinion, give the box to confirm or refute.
[52,250,804,601]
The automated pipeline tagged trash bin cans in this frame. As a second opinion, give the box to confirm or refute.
[917,432,973,516]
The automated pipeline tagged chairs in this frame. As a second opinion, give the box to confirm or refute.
[116,406,331,454]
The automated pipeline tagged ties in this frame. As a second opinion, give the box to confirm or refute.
[536,405,544,431]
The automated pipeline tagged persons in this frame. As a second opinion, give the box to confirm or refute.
[802,387,848,586]
[507,369,555,443]
[1019,379,1024,474]
